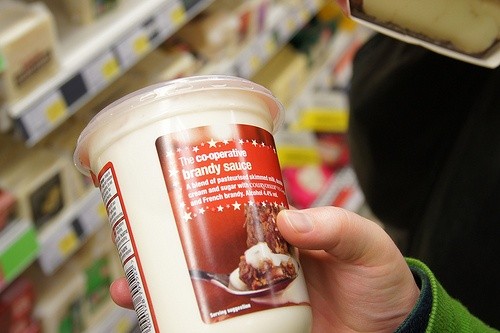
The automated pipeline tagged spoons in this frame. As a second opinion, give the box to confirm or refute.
[189,260,300,296]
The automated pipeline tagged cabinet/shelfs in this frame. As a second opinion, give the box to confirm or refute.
[0,0,388,333]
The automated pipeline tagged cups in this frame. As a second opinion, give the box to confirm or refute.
[73,74,315,333]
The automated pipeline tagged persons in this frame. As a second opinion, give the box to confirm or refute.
[109,204,500,332]
